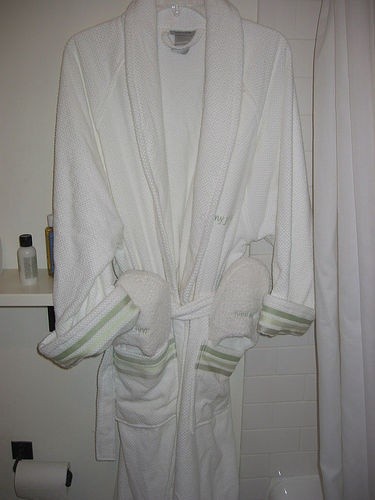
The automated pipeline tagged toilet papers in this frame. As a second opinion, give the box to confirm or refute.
[14,460,72,500]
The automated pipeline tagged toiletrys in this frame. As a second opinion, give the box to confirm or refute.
[17,214,55,287]
